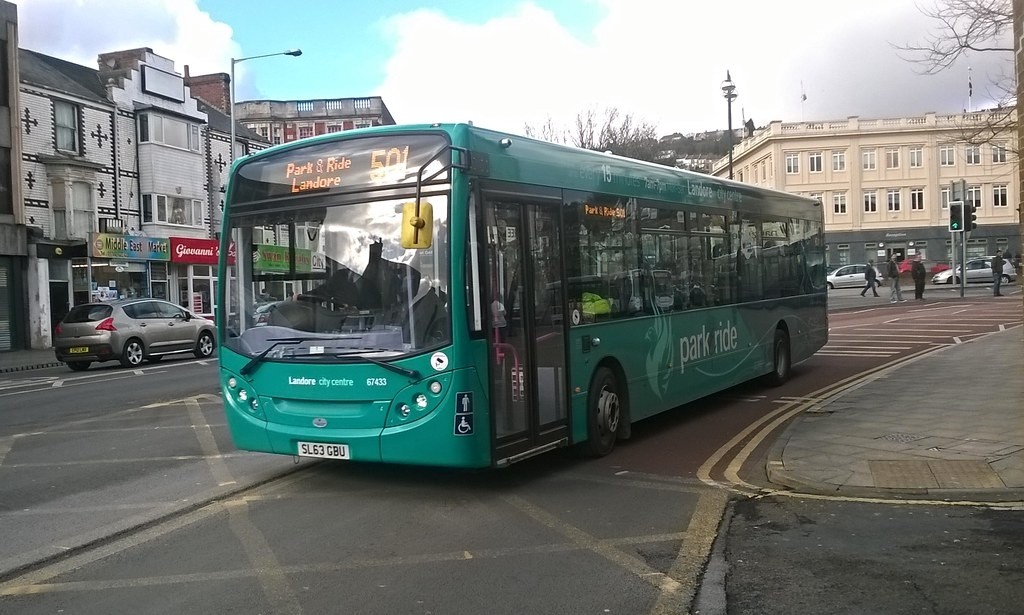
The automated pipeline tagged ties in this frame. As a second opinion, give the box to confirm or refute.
[349,272,358,283]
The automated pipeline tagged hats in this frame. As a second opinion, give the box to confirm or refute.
[891,254,897,259]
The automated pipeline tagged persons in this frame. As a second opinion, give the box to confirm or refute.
[256,289,294,303]
[292,256,397,315]
[860,249,1021,304]
[491,293,507,343]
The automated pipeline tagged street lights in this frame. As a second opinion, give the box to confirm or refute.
[231,46,302,163]
[720,69,739,181]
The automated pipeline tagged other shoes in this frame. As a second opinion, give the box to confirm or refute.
[860,294,866,297]
[891,301,897,304]
[916,298,925,301]
[899,300,907,303]
[874,295,880,297]
[994,294,1003,297]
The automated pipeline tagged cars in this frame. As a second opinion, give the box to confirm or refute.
[53,296,218,372]
[896,256,950,280]
[231,300,285,335]
[932,256,1018,286]
[827,264,885,292]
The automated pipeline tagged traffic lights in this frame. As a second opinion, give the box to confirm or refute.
[964,203,977,231]
[948,201,965,232]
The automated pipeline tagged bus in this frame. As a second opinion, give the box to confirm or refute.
[213,119,830,470]
[509,269,676,327]
